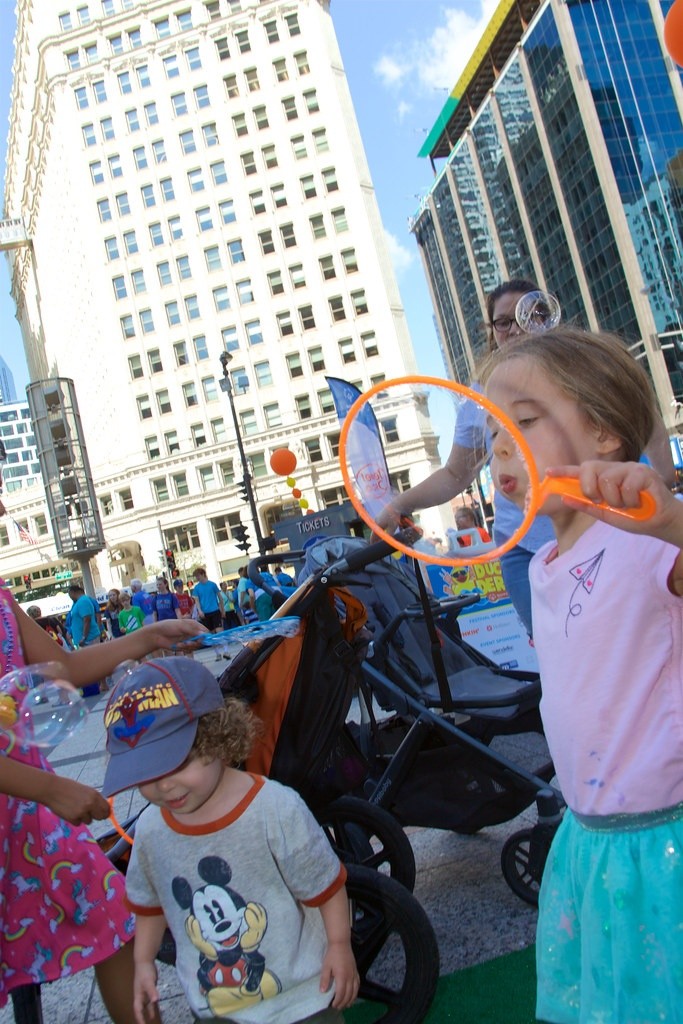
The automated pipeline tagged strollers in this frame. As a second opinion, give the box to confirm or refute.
[98,534,439,1024]
[248,530,569,912]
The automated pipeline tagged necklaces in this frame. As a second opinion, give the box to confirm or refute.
[0,605,14,697]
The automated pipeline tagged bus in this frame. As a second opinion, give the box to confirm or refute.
[18,582,163,637]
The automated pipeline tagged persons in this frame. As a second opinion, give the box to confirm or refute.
[19,558,295,707]
[453,507,492,546]
[0,440,210,1023]
[368,278,676,647]
[102,657,360,1024]
[481,329,683,1023]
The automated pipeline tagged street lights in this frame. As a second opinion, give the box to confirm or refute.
[219,351,269,573]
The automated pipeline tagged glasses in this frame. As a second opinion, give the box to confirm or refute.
[492,311,546,332]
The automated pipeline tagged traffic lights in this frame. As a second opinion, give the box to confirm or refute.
[166,549,176,569]
[233,526,251,551]
[237,479,249,502]
[24,575,31,588]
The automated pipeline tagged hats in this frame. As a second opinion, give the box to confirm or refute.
[98,656,227,797]
[173,579,183,586]
